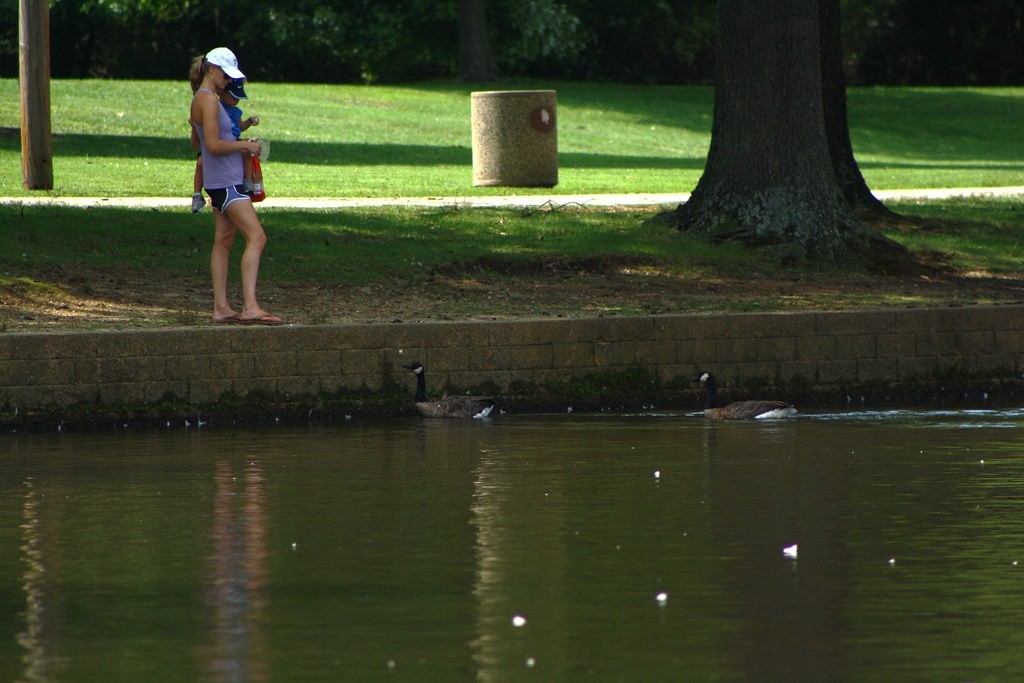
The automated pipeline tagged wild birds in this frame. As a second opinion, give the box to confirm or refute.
[402,360,498,417]
[693,371,797,420]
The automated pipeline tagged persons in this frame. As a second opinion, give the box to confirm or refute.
[189,47,284,323]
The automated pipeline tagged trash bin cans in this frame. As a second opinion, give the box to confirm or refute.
[470,90,559,188]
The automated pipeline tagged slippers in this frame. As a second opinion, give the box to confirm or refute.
[215,311,243,322]
[241,309,281,325]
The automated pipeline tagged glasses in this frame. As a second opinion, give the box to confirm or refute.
[216,65,231,79]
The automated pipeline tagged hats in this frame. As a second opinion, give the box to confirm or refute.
[205,47,247,79]
[227,78,249,100]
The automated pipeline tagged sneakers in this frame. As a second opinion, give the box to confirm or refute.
[244,180,261,196]
[190,195,205,213]
